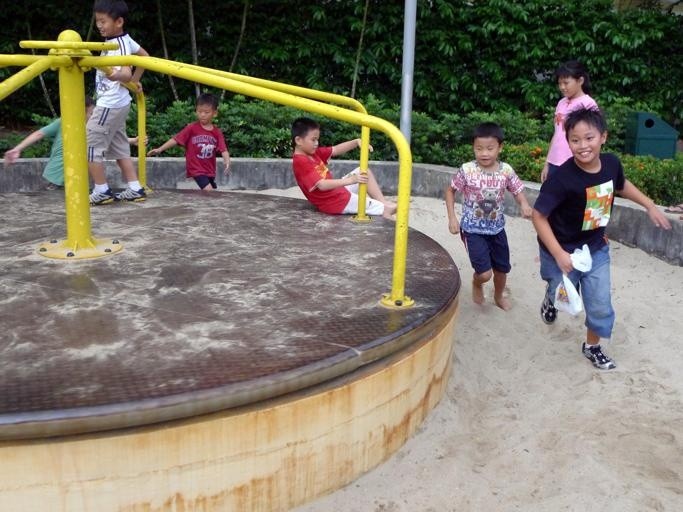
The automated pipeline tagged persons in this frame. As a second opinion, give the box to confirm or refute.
[145,91,231,191]
[84,2,150,206]
[539,62,598,185]
[532,102,672,372]
[290,116,398,220]
[444,122,534,311]
[2,96,148,192]
[662,201,682,220]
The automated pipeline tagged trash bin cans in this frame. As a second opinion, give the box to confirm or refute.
[625,112,680,158]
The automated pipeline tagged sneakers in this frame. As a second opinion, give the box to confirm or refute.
[89,188,116,205]
[540,287,559,324]
[582,342,617,370]
[114,187,147,202]
